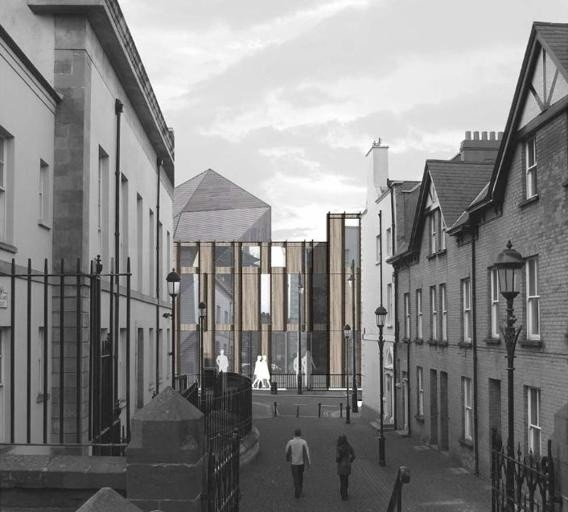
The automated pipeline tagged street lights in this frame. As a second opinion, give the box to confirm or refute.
[296,271,303,396]
[197,301,208,396]
[165,267,182,392]
[375,302,388,467]
[492,239,527,511]
[341,322,352,426]
[345,259,359,414]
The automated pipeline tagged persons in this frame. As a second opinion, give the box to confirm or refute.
[337,435,355,500]
[285,431,312,498]
